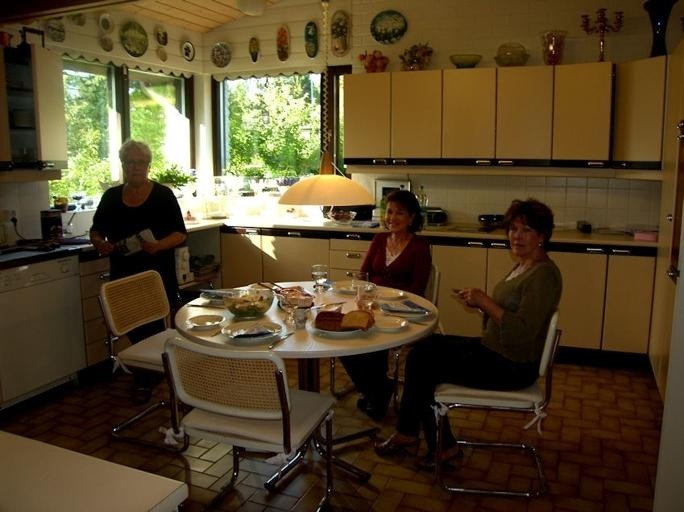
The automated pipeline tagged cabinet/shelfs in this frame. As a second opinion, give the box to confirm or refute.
[327,232,380,283]
[172,222,221,294]
[342,68,443,174]
[542,244,659,355]
[553,54,667,181]
[0,39,70,180]
[78,246,115,367]
[425,236,518,338]
[442,64,553,165]
[220,226,329,287]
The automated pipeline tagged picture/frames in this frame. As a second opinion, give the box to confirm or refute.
[373,176,411,218]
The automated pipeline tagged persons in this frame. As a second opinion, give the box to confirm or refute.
[373,197,563,475]
[336,190,432,423]
[89,138,189,405]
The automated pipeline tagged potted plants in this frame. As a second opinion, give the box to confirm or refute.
[155,164,198,198]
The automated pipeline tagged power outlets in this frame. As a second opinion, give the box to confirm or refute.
[1,209,15,223]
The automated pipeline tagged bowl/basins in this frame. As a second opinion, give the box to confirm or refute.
[447,53,483,67]
[478,213,505,230]
[492,41,531,67]
[323,208,360,225]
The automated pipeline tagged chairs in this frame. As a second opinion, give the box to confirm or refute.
[421,307,566,500]
[162,338,347,511]
[87,268,189,457]
[327,259,440,408]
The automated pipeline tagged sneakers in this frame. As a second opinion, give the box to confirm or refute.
[357,383,397,421]
[130,382,154,405]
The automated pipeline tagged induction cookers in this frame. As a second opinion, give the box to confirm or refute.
[1,238,80,270]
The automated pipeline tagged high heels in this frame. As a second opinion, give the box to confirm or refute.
[413,448,465,473]
[374,433,421,460]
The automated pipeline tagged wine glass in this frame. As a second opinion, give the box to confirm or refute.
[351,271,369,304]
[311,264,330,290]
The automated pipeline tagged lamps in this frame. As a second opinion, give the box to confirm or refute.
[276,1,378,208]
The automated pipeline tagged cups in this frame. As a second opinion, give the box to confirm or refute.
[54,196,69,213]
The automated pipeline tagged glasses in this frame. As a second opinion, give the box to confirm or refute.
[122,160,149,168]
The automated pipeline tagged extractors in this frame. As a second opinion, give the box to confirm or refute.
[0,161,64,184]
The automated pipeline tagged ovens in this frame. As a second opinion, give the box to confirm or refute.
[0,254,89,411]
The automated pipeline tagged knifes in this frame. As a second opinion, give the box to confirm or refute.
[268,332,296,351]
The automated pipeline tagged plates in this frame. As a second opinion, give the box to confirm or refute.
[153,23,170,64]
[119,18,150,58]
[184,279,431,345]
[45,19,66,44]
[368,8,410,46]
[68,12,89,27]
[177,38,197,64]
[209,40,234,70]
[11,108,35,127]
[97,12,117,54]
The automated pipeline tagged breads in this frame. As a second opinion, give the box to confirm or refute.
[316,310,374,331]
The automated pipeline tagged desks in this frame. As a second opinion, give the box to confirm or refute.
[0,429,191,511]
